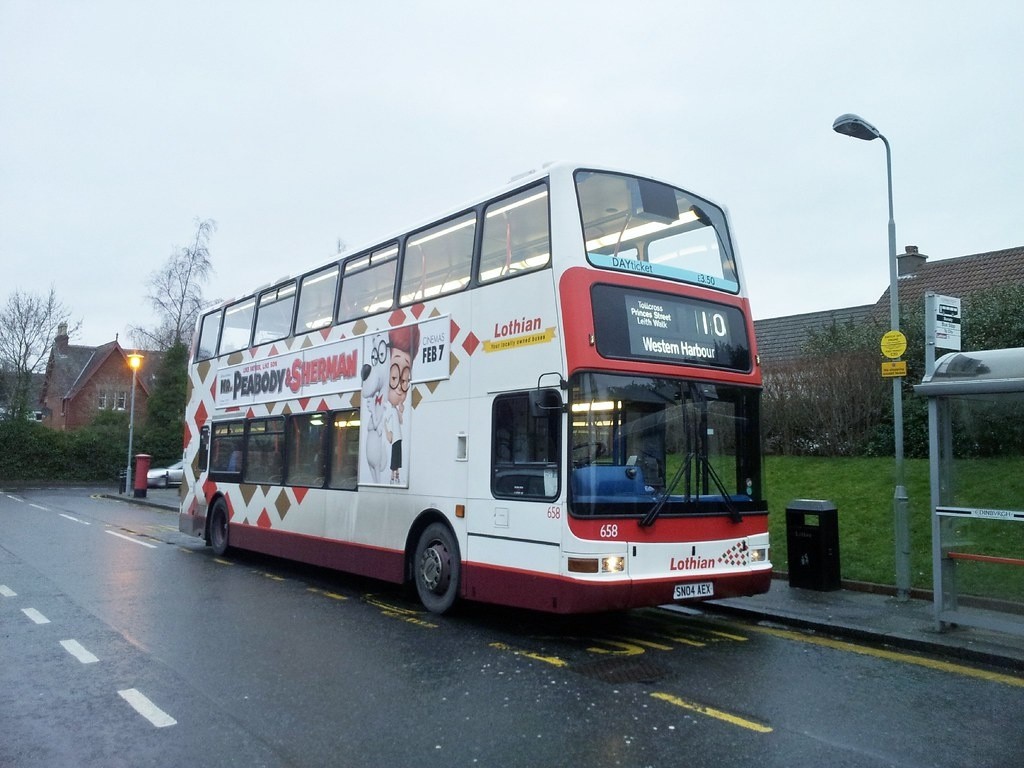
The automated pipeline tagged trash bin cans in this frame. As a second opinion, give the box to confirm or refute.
[785,498,842,592]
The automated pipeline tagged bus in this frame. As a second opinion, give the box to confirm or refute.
[175,163,773,617]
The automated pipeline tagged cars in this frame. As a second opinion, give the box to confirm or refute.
[147,460,183,486]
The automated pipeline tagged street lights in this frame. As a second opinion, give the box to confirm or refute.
[831,111,912,601]
[124,354,145,496]
